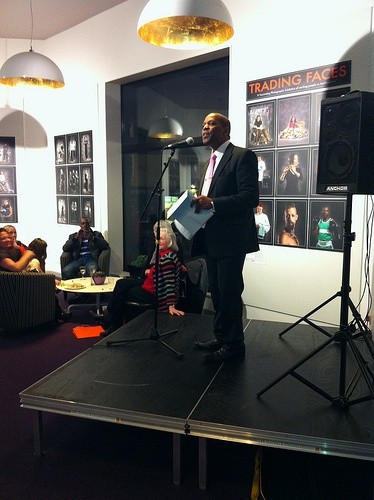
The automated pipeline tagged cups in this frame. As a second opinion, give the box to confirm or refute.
[108,278,114,289]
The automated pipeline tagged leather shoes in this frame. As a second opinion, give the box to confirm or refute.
[194,337,222,349]
[200,343,246,362]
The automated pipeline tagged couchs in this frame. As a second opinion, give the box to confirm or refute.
[117,258,208,327]
[60,247,112,278]
[0,256,56,330]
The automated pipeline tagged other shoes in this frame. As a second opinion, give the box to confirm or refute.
[68,295,87,304]
[101,326,122,337]
[57,311,73,321]
[89,310,111,329]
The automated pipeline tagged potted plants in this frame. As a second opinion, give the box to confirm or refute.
[94,271,106,285]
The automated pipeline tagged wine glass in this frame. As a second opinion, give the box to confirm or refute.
[90,265,96,276]
[80,266,85,278]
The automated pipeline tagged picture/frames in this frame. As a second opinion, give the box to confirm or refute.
[53,130,95,227]
[246,87,351,253]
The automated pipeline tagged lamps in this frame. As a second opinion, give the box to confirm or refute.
[137,0,235,51]
[147,75,183,139]
[0,0,65,88]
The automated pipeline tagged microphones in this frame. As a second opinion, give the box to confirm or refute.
[165,136,194,149]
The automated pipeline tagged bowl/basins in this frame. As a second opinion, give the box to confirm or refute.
[93,276,105,284]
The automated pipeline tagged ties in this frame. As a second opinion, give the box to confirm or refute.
[208,154,217,184]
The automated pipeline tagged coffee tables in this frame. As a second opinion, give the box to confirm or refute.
[56,276,125,319]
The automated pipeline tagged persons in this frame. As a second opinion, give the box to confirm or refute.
[0,225,36,272]
[89,220,184,338]
[0,199,13,222]
[24,238,72,320]
[280,116,309,139]
[312,205,340,249]
[254,203,270,241]
[251,115,263,141]
[279,151,303,195]
[278,203,299,246]
[62,216,109,279]
[190,112,260,362]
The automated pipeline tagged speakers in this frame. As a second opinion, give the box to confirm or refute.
[315,90,374,196]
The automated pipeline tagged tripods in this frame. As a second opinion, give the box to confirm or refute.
[254,194,374,410]
[107,150,184,358]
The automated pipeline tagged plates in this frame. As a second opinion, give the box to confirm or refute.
[64,285,86,290]
